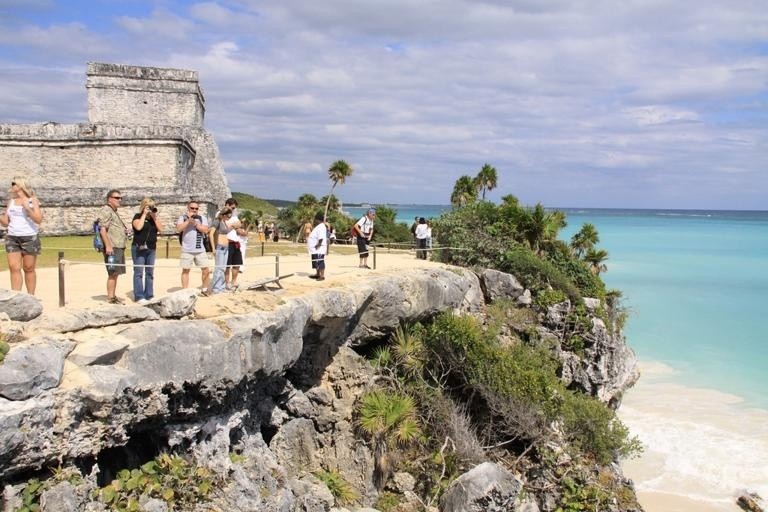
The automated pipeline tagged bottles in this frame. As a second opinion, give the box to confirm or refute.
[107,255,116,271]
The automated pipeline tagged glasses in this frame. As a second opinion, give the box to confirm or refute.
[227,215,231,219]
[190,206,199,211]
[111,197,123,200]
[231,207,235,210]
[12,182,16,185]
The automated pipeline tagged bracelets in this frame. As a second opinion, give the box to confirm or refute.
[193,221,198,227]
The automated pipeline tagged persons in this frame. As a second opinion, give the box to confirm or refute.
[175,201,210,297]
[130,198,161,303]
[414,217,428,260]
[425,222,432,256]
[215,197,243,290]
[208,205,246,294]
[97,189,129,304]
[354,207,377,270]
[237,215,359,273]
[0,175,42,296]
[306,213,327,281]
[411,216,422,259]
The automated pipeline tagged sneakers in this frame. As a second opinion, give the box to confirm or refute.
[136,298,156,304]
[309,275,325,281]
[201,291,211,297]
[107,296,125,305]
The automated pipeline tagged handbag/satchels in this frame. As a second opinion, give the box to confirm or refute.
[93,221,104,252]
[236,243,241,248]
[203,230,218,252]
[139,245,148,251]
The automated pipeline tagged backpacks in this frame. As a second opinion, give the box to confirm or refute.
[352,226,360,237]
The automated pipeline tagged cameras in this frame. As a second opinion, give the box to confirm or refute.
[149,207,157,213]
[191,214,196,219]
